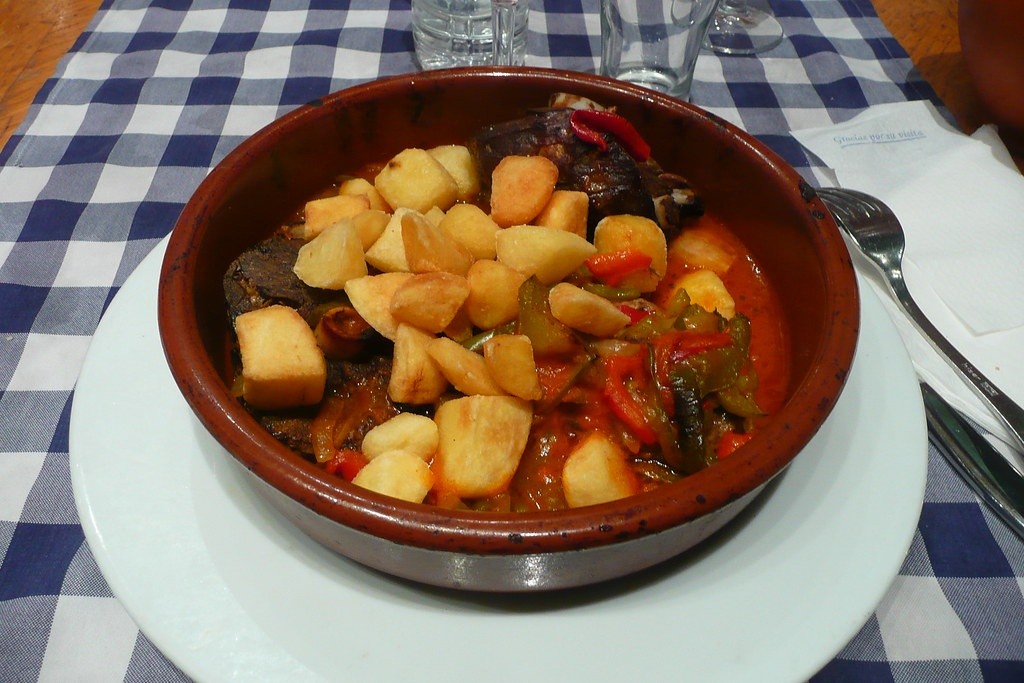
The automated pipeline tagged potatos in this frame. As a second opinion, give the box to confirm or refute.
[233,141,735,509]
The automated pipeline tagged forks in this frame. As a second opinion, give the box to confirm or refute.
[816,187,1024,449]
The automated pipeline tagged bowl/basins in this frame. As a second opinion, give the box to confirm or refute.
[158,64,859,591]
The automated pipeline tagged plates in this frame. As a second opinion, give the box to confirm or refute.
[69,230,928,683]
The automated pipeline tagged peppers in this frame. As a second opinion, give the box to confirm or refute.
[463,108,771,483]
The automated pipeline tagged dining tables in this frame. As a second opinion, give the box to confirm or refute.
[0,0,1024,683]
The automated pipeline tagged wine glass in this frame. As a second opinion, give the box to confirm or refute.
[701,0,784,56]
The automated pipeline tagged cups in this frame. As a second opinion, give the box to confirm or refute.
[600,0,720,104]
[411,0,530,71]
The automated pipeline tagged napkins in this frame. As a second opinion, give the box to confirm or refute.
[790,99,1024,478]
